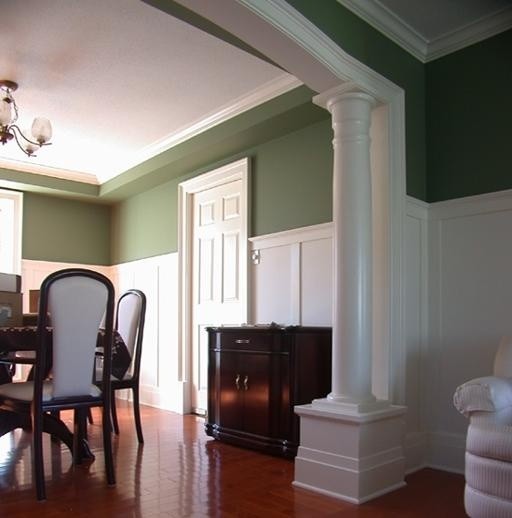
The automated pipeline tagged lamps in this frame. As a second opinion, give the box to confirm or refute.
[0,80,53,158]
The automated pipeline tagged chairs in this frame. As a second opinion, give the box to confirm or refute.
[0,268,146,503]
[452,334,512,518]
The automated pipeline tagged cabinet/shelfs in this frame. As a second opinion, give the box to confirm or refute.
[203,325,332,461]
